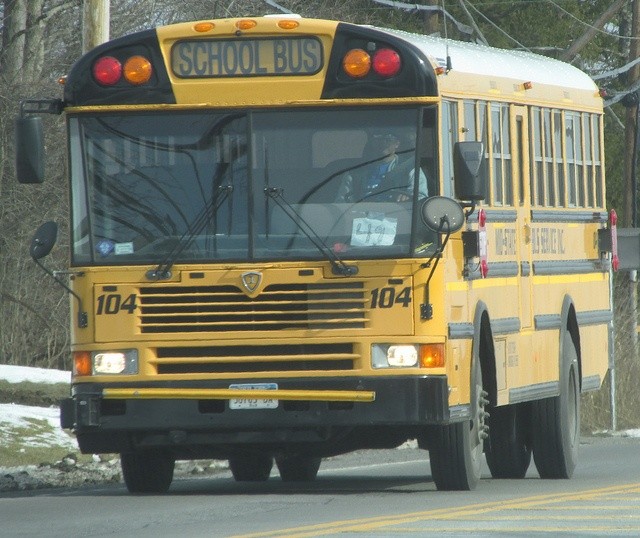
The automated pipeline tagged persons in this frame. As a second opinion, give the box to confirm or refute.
[336,124,429,203]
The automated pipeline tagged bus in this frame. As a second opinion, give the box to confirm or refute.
[18,13,614,490]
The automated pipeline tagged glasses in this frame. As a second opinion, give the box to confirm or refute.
[373,133,397,141]
